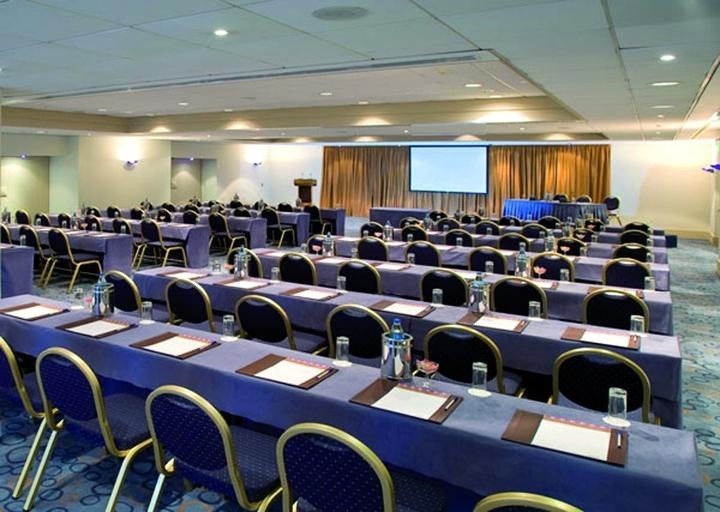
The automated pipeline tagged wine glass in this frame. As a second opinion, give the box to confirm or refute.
[417,360,440,388]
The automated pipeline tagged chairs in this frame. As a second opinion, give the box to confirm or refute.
[22,346,153,511]
[275,421,464,512]
[326,303,394,370]
[164,278,242,338]
[236,294,329,358]
[0,337,62,500]
[144,385,285,510]
[472,491,583,512]
[101,270,170,324]
[486,276,549,319]
[580,288,649,334]
[548,347,662,425]
[423,323,528,402]
[420,269,471,307]
[2,195,670,294]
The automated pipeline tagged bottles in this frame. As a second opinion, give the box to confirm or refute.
[238,244,246,255]
[97,273,107,284]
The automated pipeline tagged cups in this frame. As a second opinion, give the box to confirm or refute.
[299,212,656,280]
[471,361,488,393]
[19,236,26,247]
[607,387,629,422]
[221,315,235,337]
[335,335,350,364]
[141,301,153,323]
[195,218,200,225]
[267,264,646,338]
[19,209,128,234]
[73,288,85,308]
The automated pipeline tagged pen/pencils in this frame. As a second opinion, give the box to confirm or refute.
[259,282,270,287]
[425,307,434,312]
[331,293,340,298]
[444,397,458,411]
[634,336,637,342]
[317,369,334,378]
[521,321,525,326]
[200,341,216,351]
[199,274,211,277]
[617,431,622,448]
[116,324,136,333]
[49,309,70,316]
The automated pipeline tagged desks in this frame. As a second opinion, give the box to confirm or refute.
[133,264,685,429]
[249,245,673,337]
[1,293,705,509]
